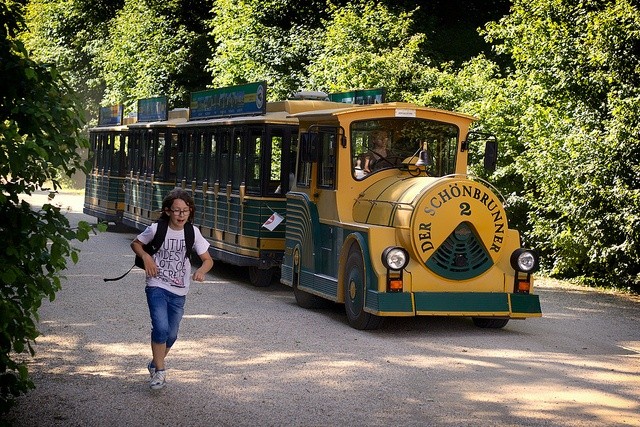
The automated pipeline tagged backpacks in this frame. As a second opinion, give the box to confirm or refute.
[103,212,195,282]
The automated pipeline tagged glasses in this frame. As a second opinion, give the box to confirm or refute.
[170,207,190,215]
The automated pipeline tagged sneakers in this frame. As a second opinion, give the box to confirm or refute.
[147,361,155,376]
[149,370,166,389]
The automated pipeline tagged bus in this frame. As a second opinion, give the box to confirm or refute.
[82,90,543,330]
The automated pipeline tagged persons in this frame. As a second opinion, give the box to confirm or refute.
[131,189,213,390]
[356,129,396,169]
